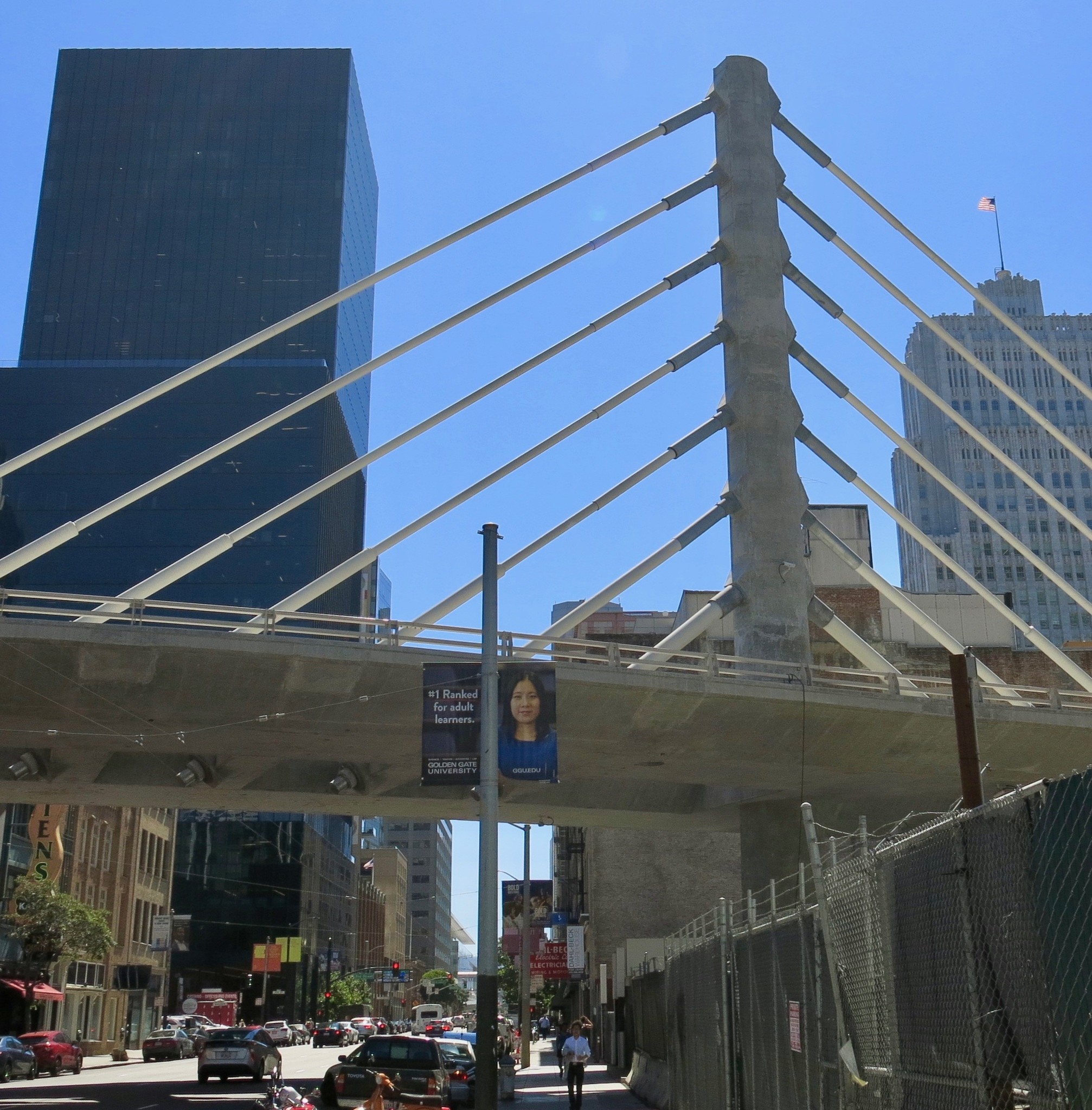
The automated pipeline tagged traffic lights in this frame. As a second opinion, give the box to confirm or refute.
[448,974,452,983]
[463,980,467,988]
[325,992,331,1004]
[401,999,406,1007]
[393,961,400,977]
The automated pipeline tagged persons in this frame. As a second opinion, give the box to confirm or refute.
[531,896,552,927]
[534,1013,595,1110]
[476,671,557,783]
[503,895,524,929]
[305,1018,315,1037]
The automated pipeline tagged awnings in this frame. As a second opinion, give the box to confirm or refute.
[0,979,64,1001]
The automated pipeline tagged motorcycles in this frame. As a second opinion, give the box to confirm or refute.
[249,1067,320,1110]
[352,1069,450,1110]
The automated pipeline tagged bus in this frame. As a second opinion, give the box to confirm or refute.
[411,1003,444,1036]
[462,1013,477,1024]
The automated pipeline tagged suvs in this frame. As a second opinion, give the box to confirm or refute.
[425,1019,445,1038]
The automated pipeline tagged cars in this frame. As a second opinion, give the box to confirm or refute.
[516,1020,540,1040]
[442,1031,477,1053]
[441,1015,465,1031]
[465,1013,516,1056]
[0,1030,85,1082]
[312,1017,412,1048]
[197,1026,282,1084]
[264,1019,312,1047]
[142,1014,230,1062]
[319,1033,475,1110]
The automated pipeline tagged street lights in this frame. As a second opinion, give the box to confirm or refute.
[364,939,388,970]
[283,916,322,1018]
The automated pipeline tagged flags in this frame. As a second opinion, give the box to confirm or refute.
[977,196,996,213]
